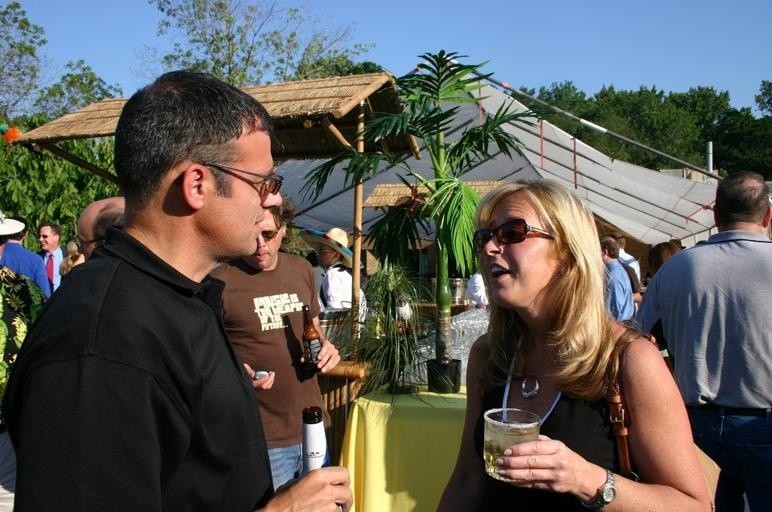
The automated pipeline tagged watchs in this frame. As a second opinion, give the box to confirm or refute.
[582,469,616,512]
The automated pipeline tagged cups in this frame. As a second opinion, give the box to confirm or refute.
[484,408,541,482]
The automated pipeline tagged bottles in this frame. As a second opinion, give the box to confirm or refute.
[303,406,330,478]
[302,305,321,371]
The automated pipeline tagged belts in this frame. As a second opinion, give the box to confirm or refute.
[701,405,765,416]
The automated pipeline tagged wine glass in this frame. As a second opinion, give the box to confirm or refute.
[450,306,491,351]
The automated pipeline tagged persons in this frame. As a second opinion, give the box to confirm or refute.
[32,221,68,298]
[435,178,710,511]
[58,241,85,277]
[315,227,367,341]
[601,171,771,511]
[61,196,127,285]
[1,69,353,512]
[304,252,325,313]
[207,187,341,498]
[453,271,487,312]
[0,214,51,298]
[0,214,52,512]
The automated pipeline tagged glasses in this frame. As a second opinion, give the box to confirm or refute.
[473,219,553,252]
[202,161,283,195]
[262,225,281,241]
[319,247,332,252]
[75,237,97,252]
[39,234,48,238]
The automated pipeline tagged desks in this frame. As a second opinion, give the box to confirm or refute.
[337,382,467,512]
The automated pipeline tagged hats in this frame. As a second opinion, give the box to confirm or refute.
[0,211,26,236]
[300,228,364,269]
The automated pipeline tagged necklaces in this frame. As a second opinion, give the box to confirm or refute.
[520,356,555,399]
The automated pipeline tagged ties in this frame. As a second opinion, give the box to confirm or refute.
[46,255,53,283]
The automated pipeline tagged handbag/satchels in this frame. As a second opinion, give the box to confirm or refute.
[692,442,721,511]
